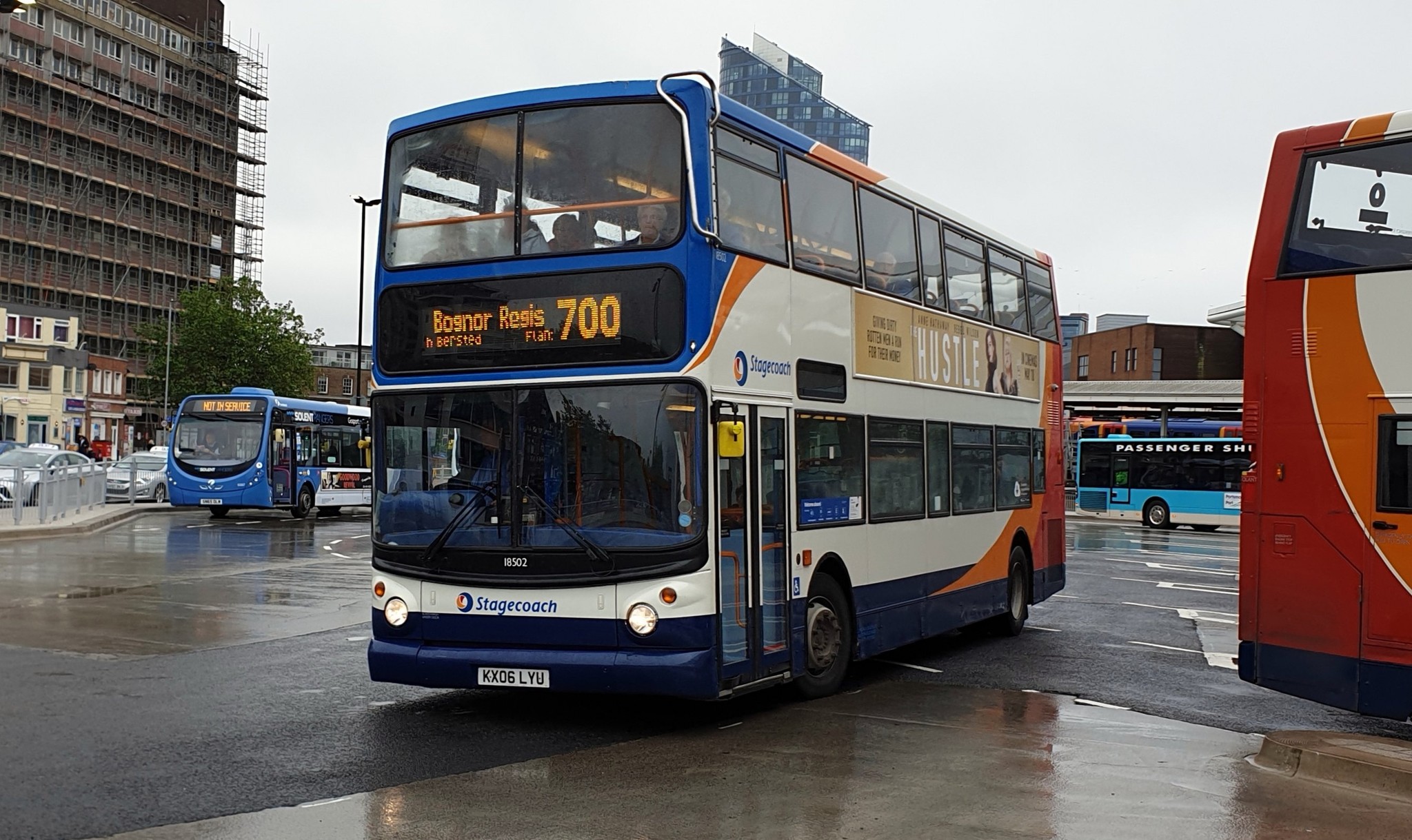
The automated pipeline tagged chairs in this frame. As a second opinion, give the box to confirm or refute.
[796,244,1028,327]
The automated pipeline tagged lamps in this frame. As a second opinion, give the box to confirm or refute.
[58,348,65,352]
[55,420,59,426]
[21,419,24,425]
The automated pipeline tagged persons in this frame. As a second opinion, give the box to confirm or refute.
[718,186,749,251]
[76,433,90,456]
[195,433,221,456]
[467,417,531,506]
[147,439,155,452]
[622,194,673,247]
[420,202,589,264]
[985,328,1018,397]
[977,466,1006,508]
[871,252,911,296]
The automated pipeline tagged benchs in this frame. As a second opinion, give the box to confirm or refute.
[1288,238,1409,274]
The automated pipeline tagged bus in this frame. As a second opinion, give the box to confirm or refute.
[1229,106,1411,723]
[164,386,374,520]
[1074,433,1244,533]
[366,70,1071,713]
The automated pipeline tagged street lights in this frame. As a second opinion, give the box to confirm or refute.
[163,299,182,450]
[353,195,383,405]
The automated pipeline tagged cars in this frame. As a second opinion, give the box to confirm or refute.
[104,446,183,503]
[0,443,107,506]
[0,440,28,454]
